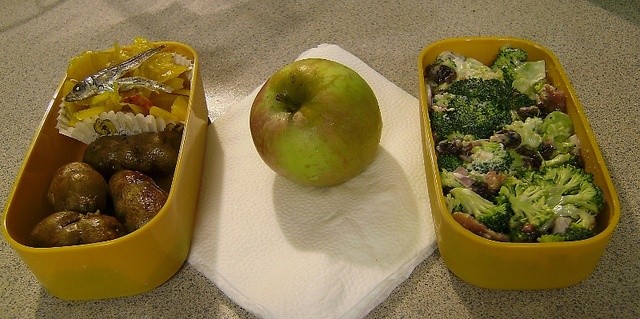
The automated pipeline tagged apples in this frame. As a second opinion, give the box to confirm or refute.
[249,57,383,189]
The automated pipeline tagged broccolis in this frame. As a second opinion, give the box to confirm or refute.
[424,44,606,243]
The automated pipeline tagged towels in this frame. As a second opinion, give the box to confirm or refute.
[188,43,438,319]
